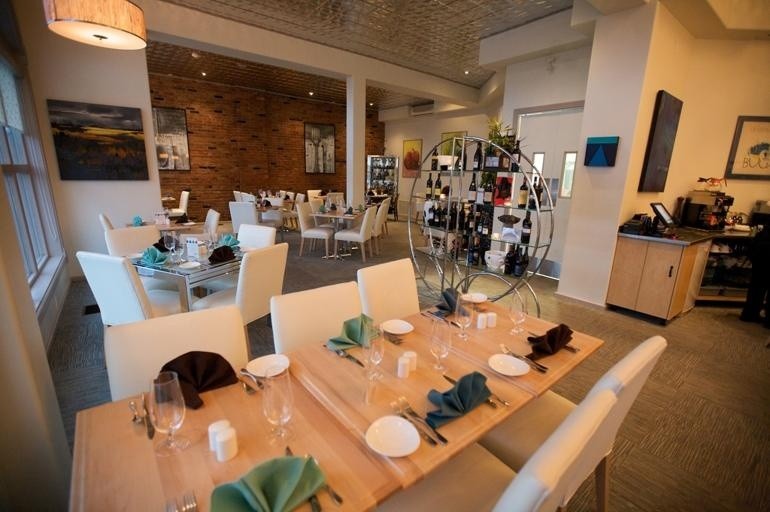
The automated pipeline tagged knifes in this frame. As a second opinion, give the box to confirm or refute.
[443,375,498,412]
[284,445,326,511]
[527,329,581,355]
[420,309,458,328]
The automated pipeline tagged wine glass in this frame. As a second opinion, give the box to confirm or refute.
[426,317,454,370]
[453,292,473,342]
[262,367,294,440]
[163,225,215,267]
[360,320,388,381]
[147,371,192,459]
[508,292,530,335]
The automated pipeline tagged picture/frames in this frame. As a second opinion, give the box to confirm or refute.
[304,122,336,174]
[724,115,770,181]
[650,202,674,226]
[402,139,423,178]
[152,106,191,172]
[441,131,467,156]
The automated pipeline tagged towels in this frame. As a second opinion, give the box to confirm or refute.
[210,455,325,512]
[432,287,461,318]
[152,350,238,410]
[326,312,378,352]
[425,371,492,429]
[525,322,573,361]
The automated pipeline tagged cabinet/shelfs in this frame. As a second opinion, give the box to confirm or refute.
[412,168,556,278]
[605,221,770,327]
[366,155,399,221]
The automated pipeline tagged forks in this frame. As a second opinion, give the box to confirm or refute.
[233,366,266,395]
[165,490,199,512]
[386,398,438,448]
[498,343,554,375]
[399,396,449,447]
[325,342,367,367]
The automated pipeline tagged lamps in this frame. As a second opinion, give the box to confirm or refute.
[43,0,148,51]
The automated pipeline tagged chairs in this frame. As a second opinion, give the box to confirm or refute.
[365,386,620,512]
[356,257,420,325]
[270,281,363,355]
[479,334,668,512]
[103,305,249,403]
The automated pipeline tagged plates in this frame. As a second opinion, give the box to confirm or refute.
[381,319,415,335]
[366,416,420,458]
[461,294,487,303]
[489,354,531,375]
[245,354,291,379]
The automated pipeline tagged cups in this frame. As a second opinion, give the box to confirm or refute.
[476,313,488,330]
[397,352,418,378]
[207,420,238,463]
[487,312,498,329]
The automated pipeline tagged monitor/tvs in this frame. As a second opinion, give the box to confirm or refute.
[644,203,676,237]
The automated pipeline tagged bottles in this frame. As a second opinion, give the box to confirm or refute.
[423,137,544,279]
